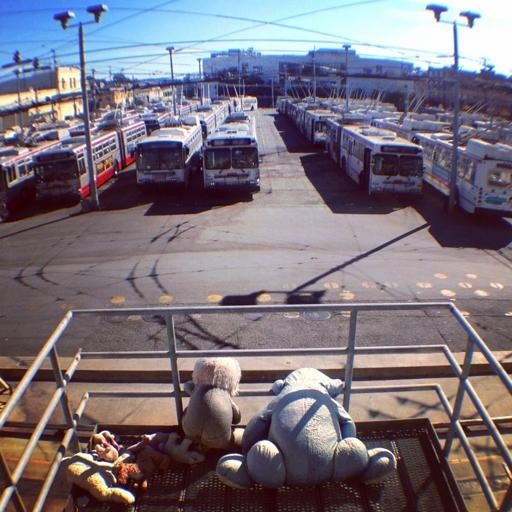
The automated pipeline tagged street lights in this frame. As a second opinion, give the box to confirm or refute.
[166,46,177,116]
[425,3,481,217]
[53,3,108,212]
[342,43,352,110]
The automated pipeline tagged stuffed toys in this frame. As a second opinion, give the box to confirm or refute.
[40,430,164,508]
[158,433,205,470]
[215,368,398,490]
[181,357,246,451]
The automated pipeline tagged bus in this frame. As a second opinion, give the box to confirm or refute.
[1,94,261,205]
[275,94,512,219]
